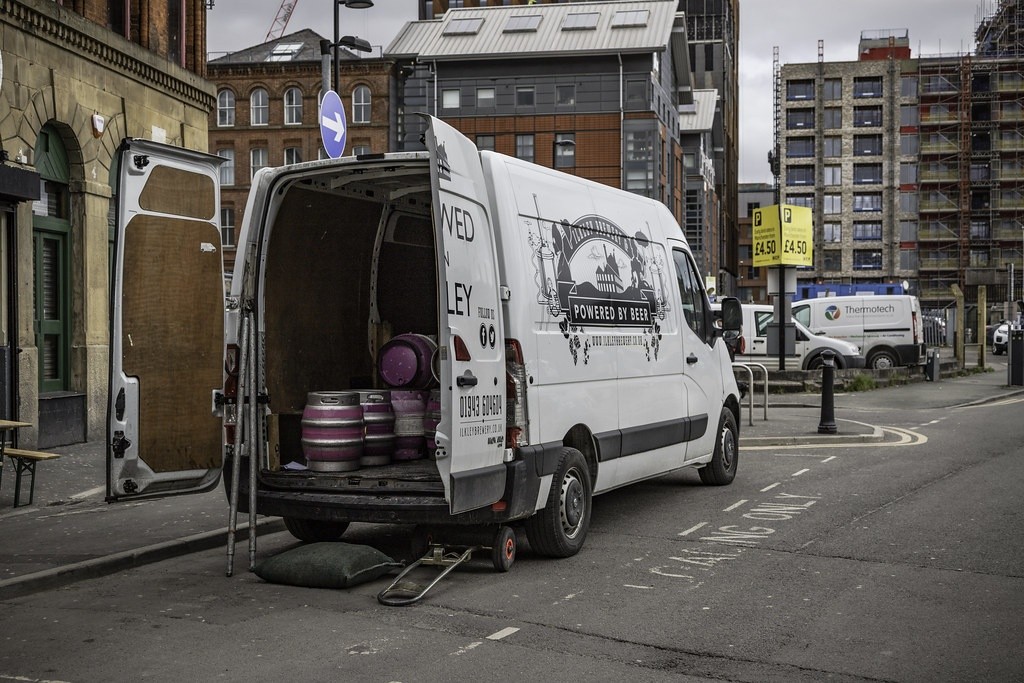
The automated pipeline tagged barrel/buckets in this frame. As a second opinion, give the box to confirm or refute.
[300,330,440,474]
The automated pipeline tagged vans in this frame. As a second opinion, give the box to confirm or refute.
[790,294,925,379]
[103,112,744,557]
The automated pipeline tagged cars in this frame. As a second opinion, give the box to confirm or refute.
[921,315,972,349]
[681,302,866,392]
[992,323,1022,356]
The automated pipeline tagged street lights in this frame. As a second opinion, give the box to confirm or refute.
[332,0,376,94]
[319,36,374,161]
